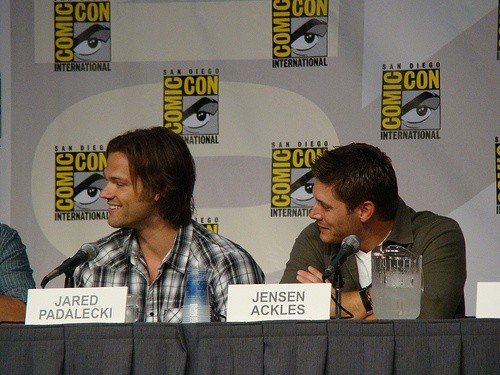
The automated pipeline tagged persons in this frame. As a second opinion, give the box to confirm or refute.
[0,223,38,322]
[64,126,265,322]
[269,143,467,319]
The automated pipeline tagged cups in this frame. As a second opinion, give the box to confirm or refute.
[370,251,422,320]
[181,268,210,323]
[125,294,138,323]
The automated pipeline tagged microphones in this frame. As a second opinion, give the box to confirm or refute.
[40,242,99,288]
[322,235,361,279]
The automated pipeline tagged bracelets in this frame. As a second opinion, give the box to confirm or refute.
[359,288,374,316]
